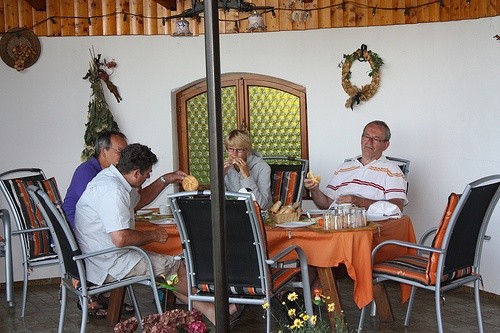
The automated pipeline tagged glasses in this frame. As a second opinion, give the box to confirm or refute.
[106,146,124,155]
[362,134,388,144]
[226,146,246,153]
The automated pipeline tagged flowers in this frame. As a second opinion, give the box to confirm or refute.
[114,309,209,333]
[260,288,349,333]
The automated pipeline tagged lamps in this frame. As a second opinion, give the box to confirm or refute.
[166,0,275,36]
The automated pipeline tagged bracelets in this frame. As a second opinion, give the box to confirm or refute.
[160,176,168,185]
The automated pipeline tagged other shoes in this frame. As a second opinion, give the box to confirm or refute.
[283,293,304,315]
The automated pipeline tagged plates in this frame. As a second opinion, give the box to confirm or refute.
[275,221,317,230]
[149,218,176,225]
[302,209,326,214]
[367,214,389,221]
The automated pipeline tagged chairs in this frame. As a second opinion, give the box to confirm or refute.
[344,157,410,201]
[0,168,64,319]
[356,172,500,333]
[166,190,316,333]
[27,185,164,333]
[261,155,309,206]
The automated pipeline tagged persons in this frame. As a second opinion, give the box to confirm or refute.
[224,130,274,210]
[51,131,245,329]
[282,121,408,326]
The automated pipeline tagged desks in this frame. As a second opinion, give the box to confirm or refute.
[134,201,419,333]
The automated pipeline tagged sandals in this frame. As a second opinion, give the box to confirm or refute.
[98,292,133,314]
[77,299,107,319]
[228,302,245,331]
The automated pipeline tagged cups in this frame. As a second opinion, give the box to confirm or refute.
[159,205,172,215]
[137,210,152,219]
[321,204,367,230]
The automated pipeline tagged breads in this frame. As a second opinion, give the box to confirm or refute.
[182,176,199,191]
[269,201,301,214]
[229,156,236,164]
[307,170,321,184]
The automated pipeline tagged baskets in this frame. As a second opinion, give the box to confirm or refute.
[269,211,303,224]
